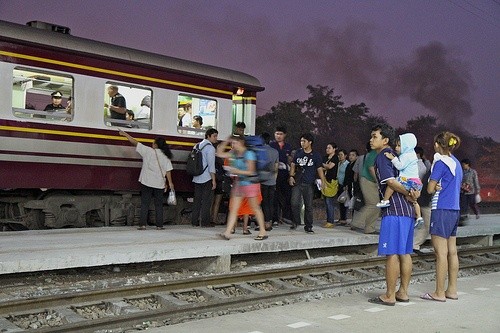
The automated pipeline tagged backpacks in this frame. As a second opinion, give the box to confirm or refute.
[186,143,212,177]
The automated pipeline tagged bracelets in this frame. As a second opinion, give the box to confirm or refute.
[289,175,295,178]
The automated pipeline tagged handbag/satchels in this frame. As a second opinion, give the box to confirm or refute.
[290,169,306,186]
[338,187,365,211]
[320,178,339,198]
[167,189,178,206]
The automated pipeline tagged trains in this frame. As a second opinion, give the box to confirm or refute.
[0,19,265,231]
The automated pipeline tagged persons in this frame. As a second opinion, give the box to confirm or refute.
[103,86,127,126]
[376,133,425,229]
[192,116,203,129]
[117,127,174,231]
[335,149,351,225]
[350,145,380,234]
[368,125,420,306]
[125,108,134,120]
[322,143,339,228]
[191,129,219,228]
[43,91,66,118]
[177,104,191,128]
[342,150,360,227]
[210,122,279,240]
[420,132,463,302]
[268,126,293,228]
[290,133,327,234]
[461,159,480,220]
[414,145,433,254]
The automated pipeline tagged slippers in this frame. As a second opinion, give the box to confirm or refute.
[368,295,396,307]
[216,234,230,240]
[421,292,459,302]
[254,235,269,240]
[242,229,252,235]
[231,229,236,234]
[395,296,411,302]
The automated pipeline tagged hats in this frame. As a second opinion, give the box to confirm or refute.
[51,90,64,98]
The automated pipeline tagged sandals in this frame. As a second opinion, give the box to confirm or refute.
[376,199,391,208]
[413,217,425,228]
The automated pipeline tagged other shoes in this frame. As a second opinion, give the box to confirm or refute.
[265,226,273,231]
[254,228,260,231]
[192,223,216,228]
[321,221,335,228]
[290,223,297,230]
[272,220,291,228]
[138,225,147,230]
[157,226,166,231]
[304,227,314,234]
[336,219,347,225]
